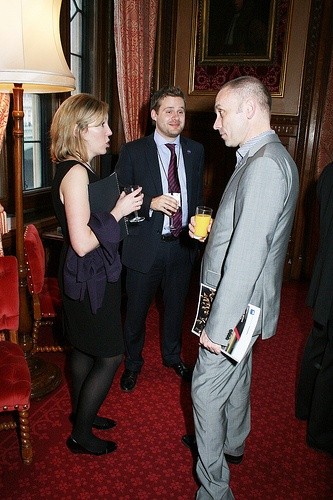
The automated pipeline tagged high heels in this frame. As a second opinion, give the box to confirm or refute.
[69,412,117,430]
[66,436,119,456]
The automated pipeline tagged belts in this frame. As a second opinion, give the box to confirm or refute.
[161,231,184,241]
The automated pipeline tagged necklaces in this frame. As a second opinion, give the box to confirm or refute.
[76,152,94,172]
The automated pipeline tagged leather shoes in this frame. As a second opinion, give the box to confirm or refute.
[170,361,189,377]
[120,365,141,392]
[181,435,243,464]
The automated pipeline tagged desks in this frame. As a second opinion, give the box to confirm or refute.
[42,225,65,265]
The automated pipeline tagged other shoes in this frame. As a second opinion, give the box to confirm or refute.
[308,440,333,450]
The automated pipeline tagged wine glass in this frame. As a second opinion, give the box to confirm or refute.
[124,185,145,222]
[164,207,179,230]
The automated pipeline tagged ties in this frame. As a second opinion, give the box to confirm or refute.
[166,143,182,237]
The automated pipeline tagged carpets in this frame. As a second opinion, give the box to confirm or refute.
[0,279,333,500]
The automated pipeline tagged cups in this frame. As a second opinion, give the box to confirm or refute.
[194,206,213,237]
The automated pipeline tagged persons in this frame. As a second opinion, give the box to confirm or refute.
[49,93,144,457]
[114,85,205,393]
[295,161,333,453]
[181,76,298,500]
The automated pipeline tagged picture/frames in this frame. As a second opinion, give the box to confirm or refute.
[187,0,296,98]
[154,0,179,92]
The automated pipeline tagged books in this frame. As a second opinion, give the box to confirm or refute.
[191,282,262,364]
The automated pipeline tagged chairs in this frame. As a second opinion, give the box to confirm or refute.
[22,221,75,354]
[0,255,35,466]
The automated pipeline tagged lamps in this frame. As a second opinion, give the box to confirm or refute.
[0,0,79,402]
